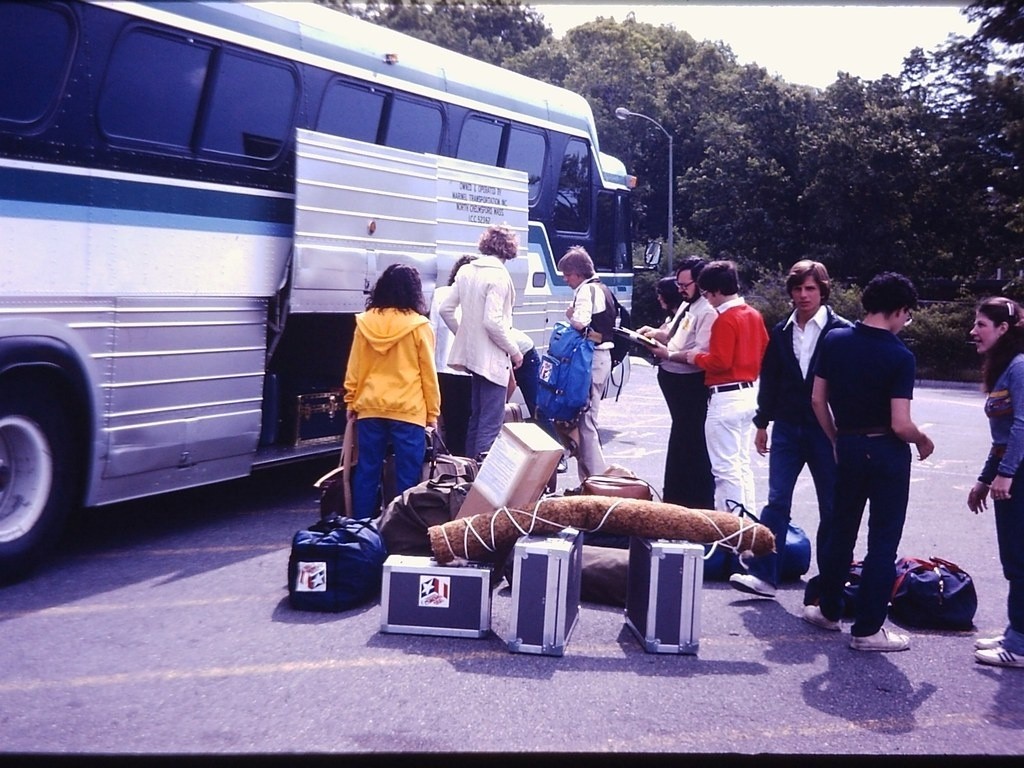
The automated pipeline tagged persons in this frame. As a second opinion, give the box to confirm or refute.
[656,275,684,316]
[635,257,715,511]
[558,247,619,497]
[429,226,541,472]
[697,260,770,524]
[344,264,441,519]
[810,272,935,651]
[967,297,1024,668]
[729,259,855,597]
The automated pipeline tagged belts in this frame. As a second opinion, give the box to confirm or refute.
[709,382,752,394]
[839,426,892,435]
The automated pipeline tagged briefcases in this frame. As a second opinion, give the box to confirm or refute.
[380,527,701,657]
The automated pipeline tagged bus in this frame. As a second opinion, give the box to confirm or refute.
[0,0,660,588]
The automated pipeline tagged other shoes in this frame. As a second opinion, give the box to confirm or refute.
[803,605,841,632]
[850,627,912,650]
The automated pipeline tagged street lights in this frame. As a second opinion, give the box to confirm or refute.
[616,108,674,277]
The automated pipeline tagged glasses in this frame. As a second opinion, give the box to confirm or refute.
[674,281,694,290]
[700,291,708,298]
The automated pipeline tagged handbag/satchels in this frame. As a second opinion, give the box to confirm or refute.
[287,427,480,613]
[583,474,652,501]
[806,559,865,617]
[706,498,811,578]
[889,556,978,627]
[609,291,635,370]
[534,286,597,422]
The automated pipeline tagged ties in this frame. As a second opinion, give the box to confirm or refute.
[654,303,690,366]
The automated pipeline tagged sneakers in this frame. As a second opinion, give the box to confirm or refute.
[730,573,776,597]
[975,635,1006,649]
[976,647,1024,668]
